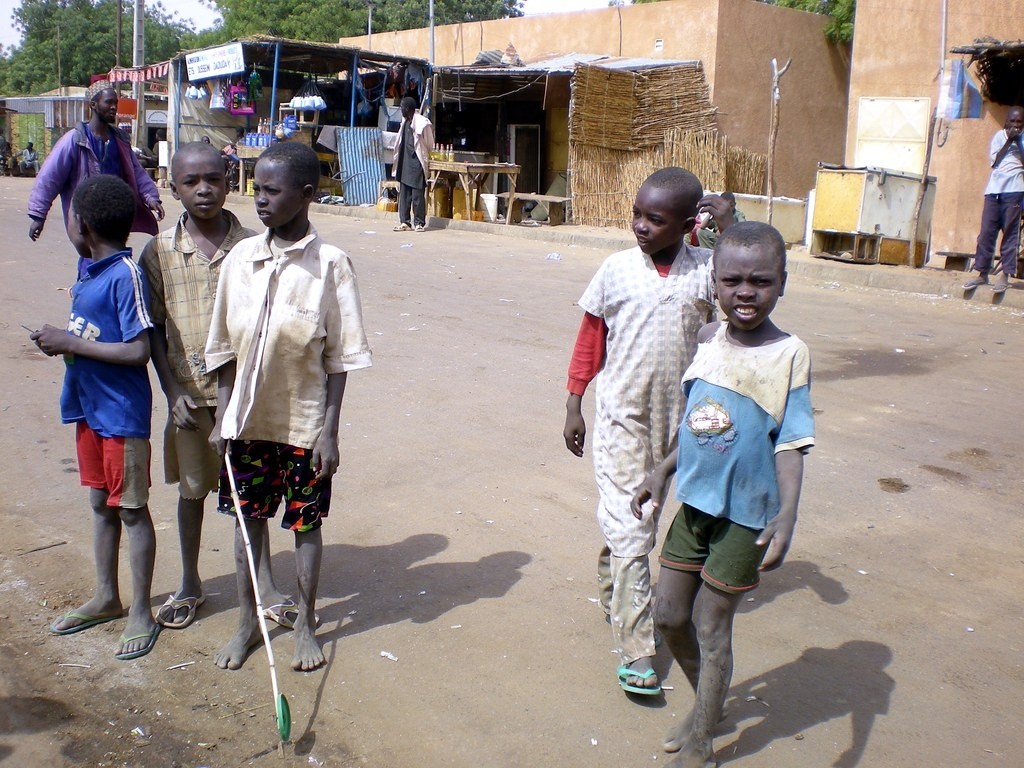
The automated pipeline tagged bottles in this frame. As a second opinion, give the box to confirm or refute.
[440,144,445,162]
[246,133,252,146]
[258,117,263,134]
[272,134,278,143]
[259,135,264,146]
[431,143,435,160]
[262,134,269,147]
[449,144,455,162]
[445,145,449,162]
[435,143,440,160]
[262,119,270,134]
[252,133,258,146]
[250,177,255,195]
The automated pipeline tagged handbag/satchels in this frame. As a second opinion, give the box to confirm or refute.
[184,63,266,116]
[290,81,327,111]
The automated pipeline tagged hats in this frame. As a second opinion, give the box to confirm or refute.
[86,79,111,100]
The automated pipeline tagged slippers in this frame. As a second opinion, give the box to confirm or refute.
[415,225,425,231]
[113,624,161,660]
[393,223,408,230]
[617,663,662,695]
[262,596,320,630]
[991,283,1012,293]
[605,613,662,646]
[157,590,205,627]
[50,607,121,633]
[963,277,986,289]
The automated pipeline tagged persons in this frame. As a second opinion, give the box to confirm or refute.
[30,176,160,661]
[392,97,434,232]
[630,220,816,768]
[138,141,319,627]
[0,129,39,176]
[139,130,165,168]
[202,126,245,171]
[565,167,737,696]
[205,141,373,670]
[27,79,165,281]
[962,108,1024,293]
[684,191,746,248]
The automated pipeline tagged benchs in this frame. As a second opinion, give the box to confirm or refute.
[936,251,1024,280]
[496,192,572,224]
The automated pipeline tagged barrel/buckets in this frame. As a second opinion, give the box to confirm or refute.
[284,114,297,129]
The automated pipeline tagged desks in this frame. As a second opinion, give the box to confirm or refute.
[425,161,520,224]
